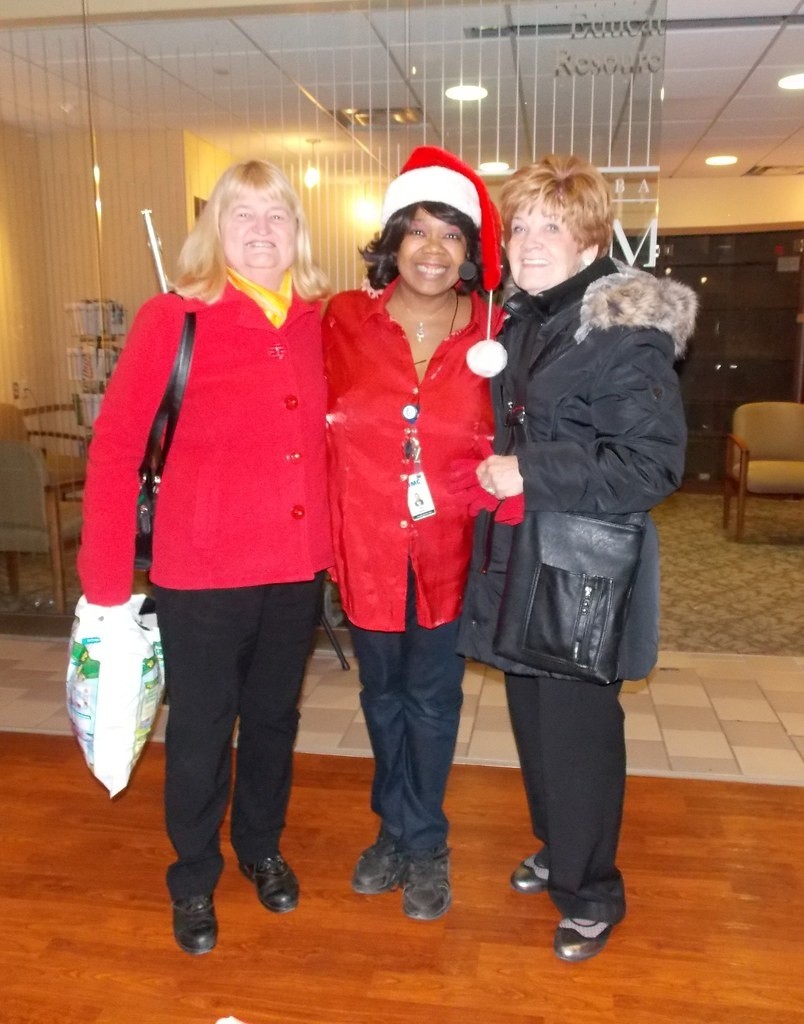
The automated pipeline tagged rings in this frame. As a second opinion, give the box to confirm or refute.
[487,488,495,495]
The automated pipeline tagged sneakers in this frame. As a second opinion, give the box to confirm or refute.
[403,845,451,920]
[350,831,404,894]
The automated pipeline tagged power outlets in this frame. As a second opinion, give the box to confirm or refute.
[21,380,31,399]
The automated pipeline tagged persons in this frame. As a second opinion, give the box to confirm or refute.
[76,159,330,959]
[321,146,512,923]
[455,151,697,968]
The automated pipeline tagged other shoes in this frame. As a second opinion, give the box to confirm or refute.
[510,854,551,894]
[553,916,612,962]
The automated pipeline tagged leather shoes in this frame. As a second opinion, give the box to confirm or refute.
[172,894,217,954]
[239,853,300,914]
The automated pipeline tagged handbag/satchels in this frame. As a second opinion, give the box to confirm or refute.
[133,468,154,569]
[492,510,646,686]
[66,594,165,800]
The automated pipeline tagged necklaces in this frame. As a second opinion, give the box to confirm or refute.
[391,286,453,345]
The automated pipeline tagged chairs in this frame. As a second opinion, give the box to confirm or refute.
[723,402,804,542]
[0,402,89,615]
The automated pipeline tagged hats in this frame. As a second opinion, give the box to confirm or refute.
[381,145,508,378]
